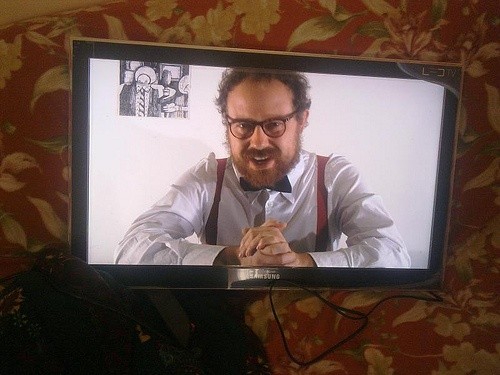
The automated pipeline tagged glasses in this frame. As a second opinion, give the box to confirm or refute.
[225,109,302,139]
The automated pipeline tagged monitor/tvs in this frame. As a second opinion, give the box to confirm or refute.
[66,35,464,294]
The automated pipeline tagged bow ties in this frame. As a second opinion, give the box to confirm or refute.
[238,172,292,193]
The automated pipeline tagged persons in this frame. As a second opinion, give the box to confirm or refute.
[113,68,412,268]
